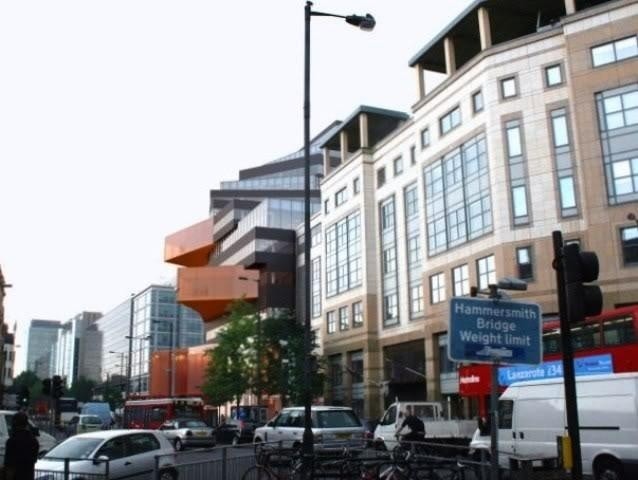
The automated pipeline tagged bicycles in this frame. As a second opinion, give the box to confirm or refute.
[239,443,473,480]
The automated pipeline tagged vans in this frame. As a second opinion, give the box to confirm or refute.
[468,372,638,480]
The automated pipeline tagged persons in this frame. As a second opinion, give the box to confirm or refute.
[0,412,41,479]
[392,406,427,452]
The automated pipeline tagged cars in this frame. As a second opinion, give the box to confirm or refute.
[252,406,365,452]
[0,397,257,480]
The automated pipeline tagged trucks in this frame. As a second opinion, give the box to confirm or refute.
[374,401,478,452]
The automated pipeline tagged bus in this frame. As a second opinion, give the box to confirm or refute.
[458,304,638,421]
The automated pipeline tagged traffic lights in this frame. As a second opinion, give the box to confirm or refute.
[52,375,64,399]
[16,391,30,405]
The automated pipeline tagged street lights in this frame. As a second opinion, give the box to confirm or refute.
[303,0,377,460]
